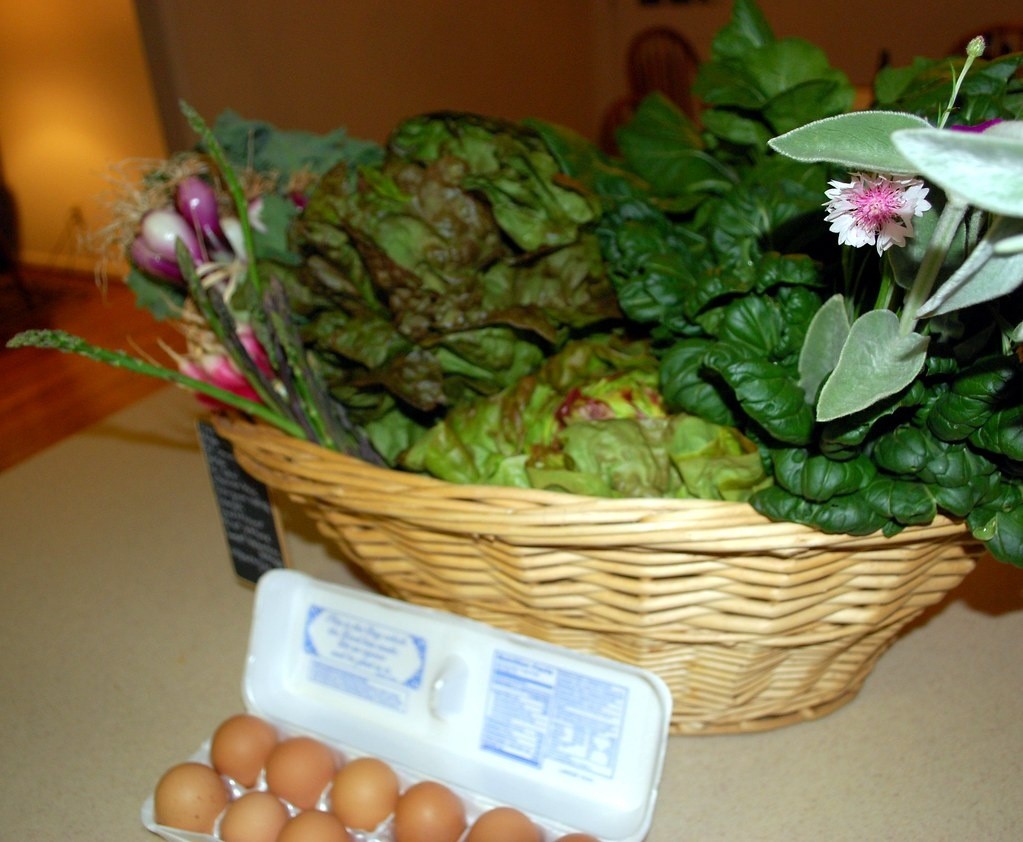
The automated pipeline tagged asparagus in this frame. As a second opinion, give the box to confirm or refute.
[5,102,369,462]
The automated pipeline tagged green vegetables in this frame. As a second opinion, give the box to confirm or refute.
[252,22,776,502]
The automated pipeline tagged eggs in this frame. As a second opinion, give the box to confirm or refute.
[153,714,609,842]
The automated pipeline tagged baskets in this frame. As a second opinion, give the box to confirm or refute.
[209,410,984,735]
